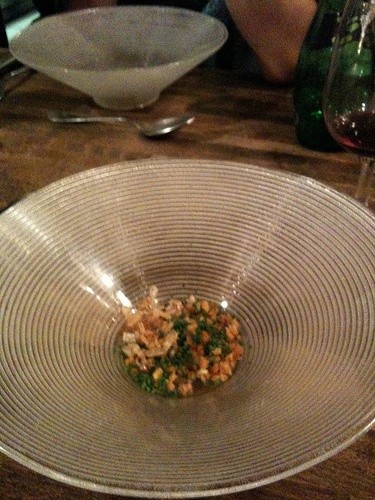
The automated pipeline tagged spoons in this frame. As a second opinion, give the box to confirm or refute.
[47,112,195,136]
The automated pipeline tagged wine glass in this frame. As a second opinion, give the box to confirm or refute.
[323,0,375,206]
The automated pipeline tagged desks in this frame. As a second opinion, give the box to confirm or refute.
[0,50,375,500]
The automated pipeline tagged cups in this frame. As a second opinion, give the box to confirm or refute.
[293,0,347,152]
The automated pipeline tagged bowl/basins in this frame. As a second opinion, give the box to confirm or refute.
[8,6,227,110]
[0,159,375,500]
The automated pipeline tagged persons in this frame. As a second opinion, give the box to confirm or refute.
[65,1,319,89]
